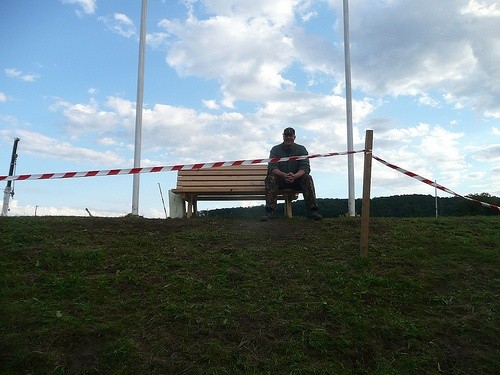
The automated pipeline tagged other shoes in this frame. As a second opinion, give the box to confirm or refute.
[308,211,322,220]
[261,208,274,222]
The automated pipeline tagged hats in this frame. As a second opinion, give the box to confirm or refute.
[283,128,295,136]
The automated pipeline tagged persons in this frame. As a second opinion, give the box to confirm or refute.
[259,127,322,223]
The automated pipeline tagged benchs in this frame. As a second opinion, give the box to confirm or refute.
[172,164,299,218]
